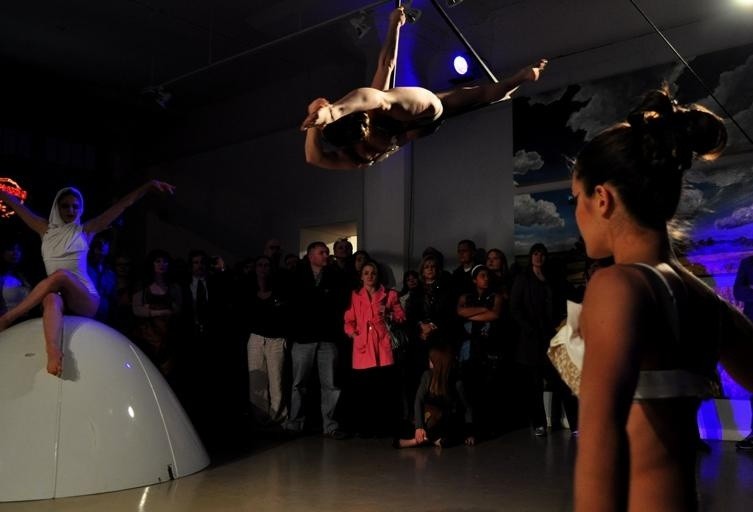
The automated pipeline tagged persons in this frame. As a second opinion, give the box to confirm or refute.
[299,5,549,173]
[2,226,713,453]
[0,179,177,375]
[545,78,752,511]
[733,254,753,451]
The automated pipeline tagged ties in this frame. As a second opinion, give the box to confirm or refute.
[196,279,207,319]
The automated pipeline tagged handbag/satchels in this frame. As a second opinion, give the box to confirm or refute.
[411,404,442,429]
[380,288,408,349]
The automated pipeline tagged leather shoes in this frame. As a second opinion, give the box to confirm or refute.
[534,426,544,435]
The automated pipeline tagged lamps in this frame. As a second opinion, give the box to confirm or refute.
[350,6,422,40]
